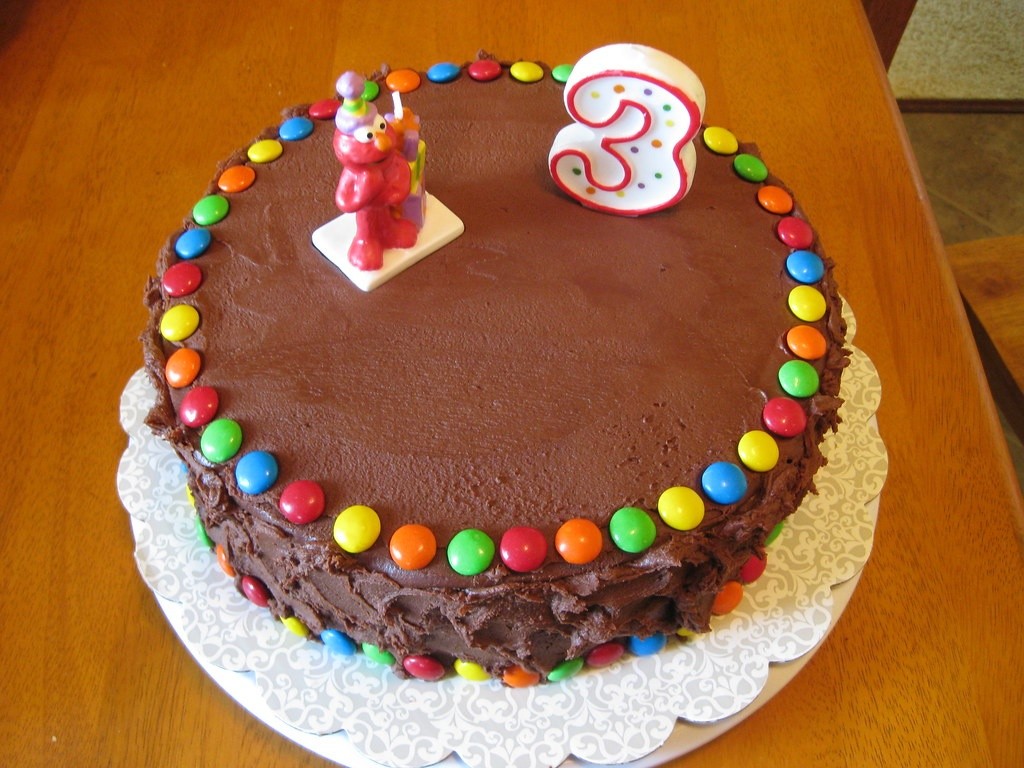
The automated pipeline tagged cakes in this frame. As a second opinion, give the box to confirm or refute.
[140,50,853,689]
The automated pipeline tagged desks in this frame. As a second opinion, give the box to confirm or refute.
[0,0,1024,768]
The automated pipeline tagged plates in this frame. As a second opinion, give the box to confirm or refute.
[129,412,882,768]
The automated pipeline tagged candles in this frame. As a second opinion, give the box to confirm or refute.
[384,91,425,230]
